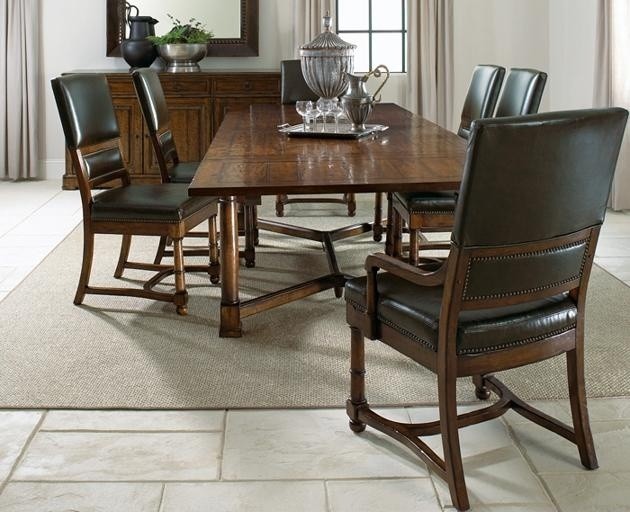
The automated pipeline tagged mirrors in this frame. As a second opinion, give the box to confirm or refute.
[106,0,258,57]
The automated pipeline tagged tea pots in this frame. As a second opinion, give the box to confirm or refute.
[341,64,390,131]
[340,142,374,184]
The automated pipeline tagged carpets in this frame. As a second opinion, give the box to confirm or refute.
[0,192,630,408]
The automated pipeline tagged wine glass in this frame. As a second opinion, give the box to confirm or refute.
[297,140,353,184]
[295,96,345,134]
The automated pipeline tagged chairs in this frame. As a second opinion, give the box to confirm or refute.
[50,73,220,316]
[342,106,630,512]
[131,69,261,268]
[374,64,505,256]
[276,59,355,217]
[391,68,547,268]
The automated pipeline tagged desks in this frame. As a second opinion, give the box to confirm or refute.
[189,102,471,339]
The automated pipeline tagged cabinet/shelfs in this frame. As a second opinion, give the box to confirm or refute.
[63,71,281,192]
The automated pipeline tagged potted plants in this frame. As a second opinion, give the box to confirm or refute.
[146,12,214,74]
